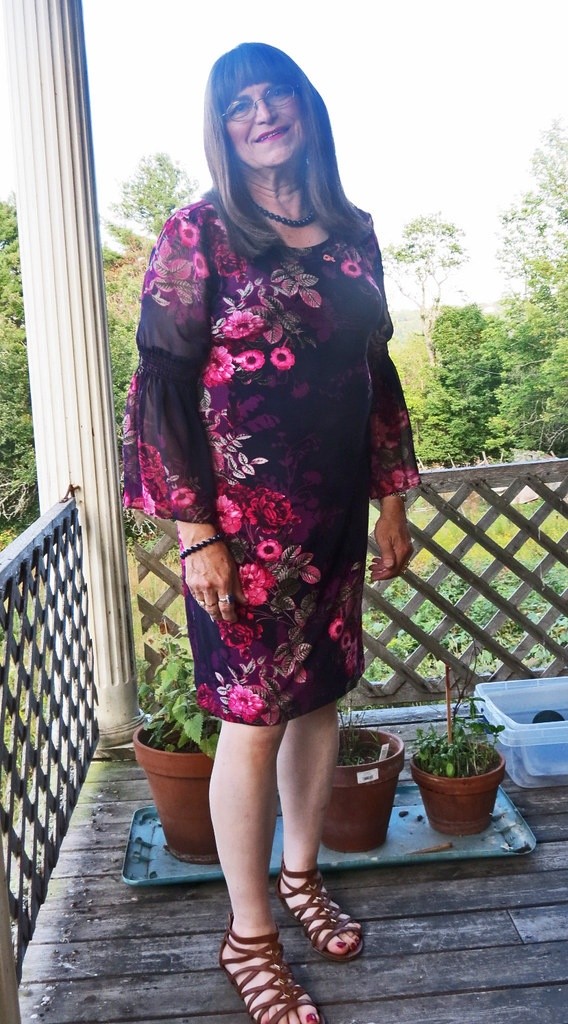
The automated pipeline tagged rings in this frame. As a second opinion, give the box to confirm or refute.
[195,599,205,607]
[219,594,234,604]
[404,560,411,568]
[401,569,407,575]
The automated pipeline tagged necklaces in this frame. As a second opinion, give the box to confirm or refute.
[252,202,316,227]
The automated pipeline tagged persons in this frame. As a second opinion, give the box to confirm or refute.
[123,43,424,1024]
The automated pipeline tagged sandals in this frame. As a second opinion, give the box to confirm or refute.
[276,853,365,963]
[219,912,325,1024]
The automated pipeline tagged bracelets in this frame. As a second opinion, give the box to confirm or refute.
[180,534,222,559]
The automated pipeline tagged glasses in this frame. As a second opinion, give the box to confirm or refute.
[220,83,300,122]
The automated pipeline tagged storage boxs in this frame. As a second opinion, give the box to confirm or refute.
[473,676,568,788]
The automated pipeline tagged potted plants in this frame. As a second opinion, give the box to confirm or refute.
[409,633,505,836]
[321,682,405,853]
[133,614,222,865]
